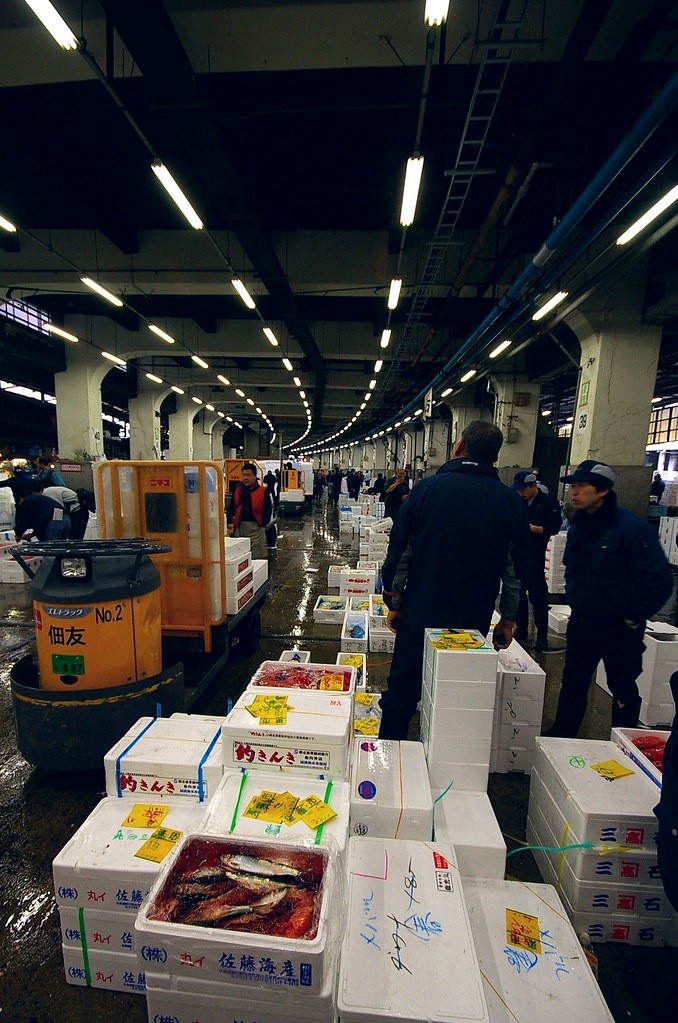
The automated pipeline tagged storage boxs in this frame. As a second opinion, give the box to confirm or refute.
[648,506,668,517]
[185,467,268,614]
[280,488,304,501]
[526,737,678,948]
[544,530,568,594]
[610,728,671,790]
[327,491,393,596]
[596,620,678,727]
[52,620,615,1023]
[546,603,572,649]
[299,462,314,495]
[485,630,546,775]
[658,516,678,567]
[83,516,99,541]
[0,487,44,583]
[313,594,396,653]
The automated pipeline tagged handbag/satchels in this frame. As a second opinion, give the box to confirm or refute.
[77,489,96,513]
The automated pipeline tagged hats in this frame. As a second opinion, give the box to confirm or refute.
[559,460,616,489]
[510,472,537,490]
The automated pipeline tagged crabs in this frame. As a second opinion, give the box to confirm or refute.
[156,851,316,938]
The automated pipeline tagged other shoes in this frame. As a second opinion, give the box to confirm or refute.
[536,643,549,656]
[513,629,528,641]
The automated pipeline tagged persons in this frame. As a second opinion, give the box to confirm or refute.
[374,468,423,521]
[650,474,665,501]
[313,467,370,505]
[298,471,306,492]
[510,471,562,651]
[378,420,530,740]
[0,457,89,541]
[227,463,272,559]
[264,469,281,507]
[540,460,672,739]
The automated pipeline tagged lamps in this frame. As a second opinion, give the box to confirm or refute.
[0,215,512,412]
[25,0,83,55]
[150,159,205,231]
[531,289,569,323]
[421,1,448,27]
[388,276,402,310]
[400,152,425,230]
[615,186,678,253]
